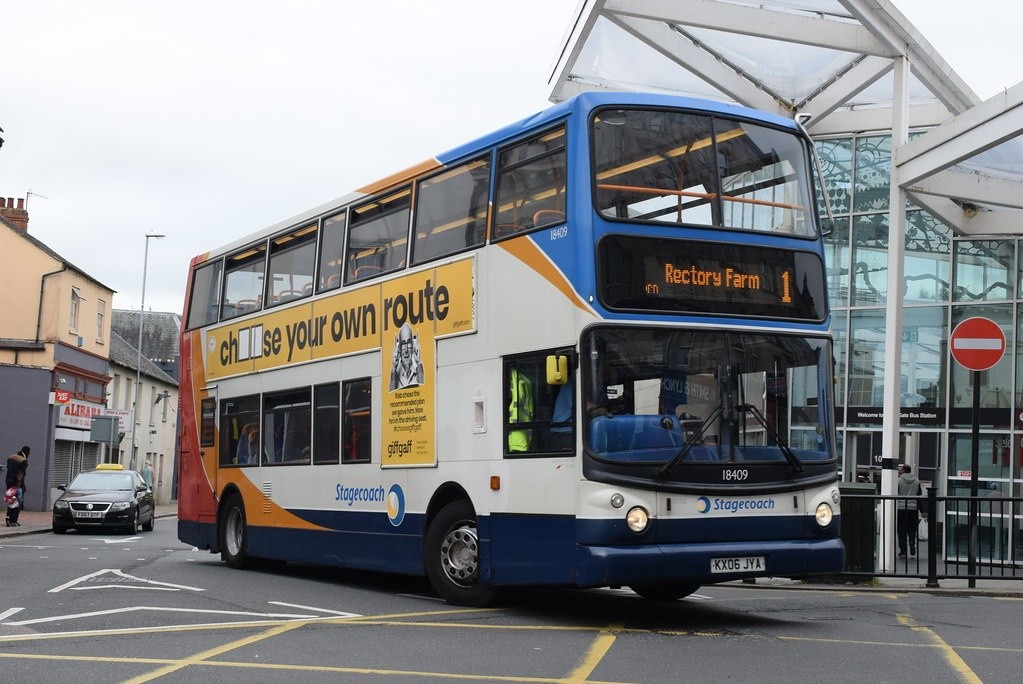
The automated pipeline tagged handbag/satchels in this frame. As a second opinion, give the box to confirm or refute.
[918,516,928,542]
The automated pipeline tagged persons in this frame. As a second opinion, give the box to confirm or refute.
[5,446,31,527]
[139,460,156,491]
[388,324,425,392]
[551,337,608,449]
[898,464,922,559]
[987,483,1005,513]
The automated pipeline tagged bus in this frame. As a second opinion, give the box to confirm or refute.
[176,92,845,607]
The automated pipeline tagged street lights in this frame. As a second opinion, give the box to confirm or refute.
[130,235,166,472]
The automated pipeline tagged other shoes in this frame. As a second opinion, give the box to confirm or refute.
[910,547,916,558]
[9,523,20,526]
[5,517,11,527]
[899,553,906,558]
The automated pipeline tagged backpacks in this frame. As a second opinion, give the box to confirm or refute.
[2,486,17,504]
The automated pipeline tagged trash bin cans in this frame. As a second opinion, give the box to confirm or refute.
[801,482,877,583]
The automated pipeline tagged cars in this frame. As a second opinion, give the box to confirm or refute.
[52,463,155,536]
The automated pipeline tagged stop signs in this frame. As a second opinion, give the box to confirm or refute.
[950,317,1006,372]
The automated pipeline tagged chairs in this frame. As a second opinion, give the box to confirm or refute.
[231,421,311,465]
[209,209,565,323]
[527,375,573,452]
